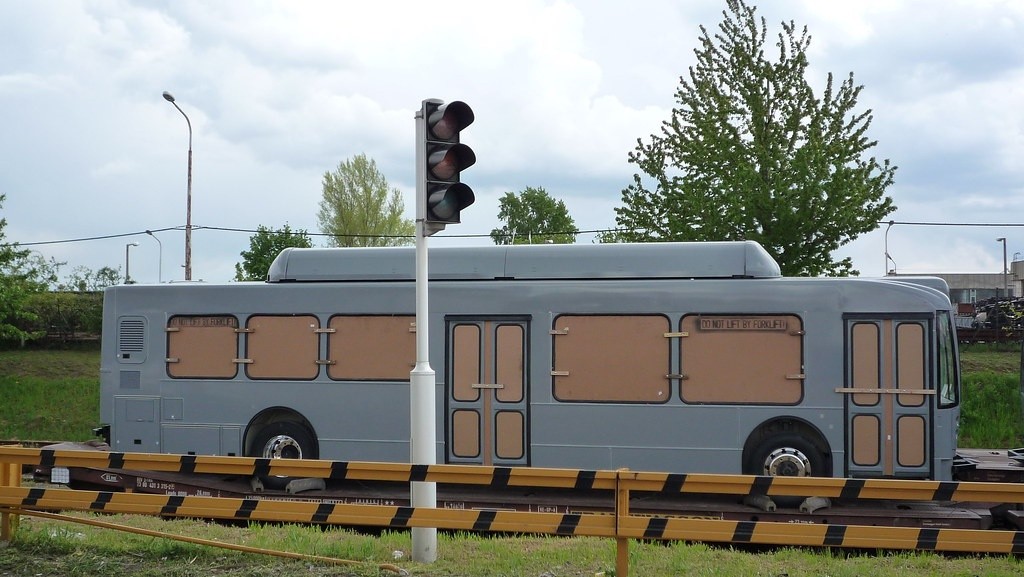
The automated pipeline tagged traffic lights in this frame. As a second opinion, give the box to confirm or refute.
[420,97,476,225]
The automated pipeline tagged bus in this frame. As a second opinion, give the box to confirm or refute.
[92,241,975,509]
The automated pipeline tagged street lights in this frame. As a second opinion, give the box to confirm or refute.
[124,242,139,285]
[884,252,896,275]
[162,91,192,282]
[885,220,895,275]
[995,236,1009,298]
